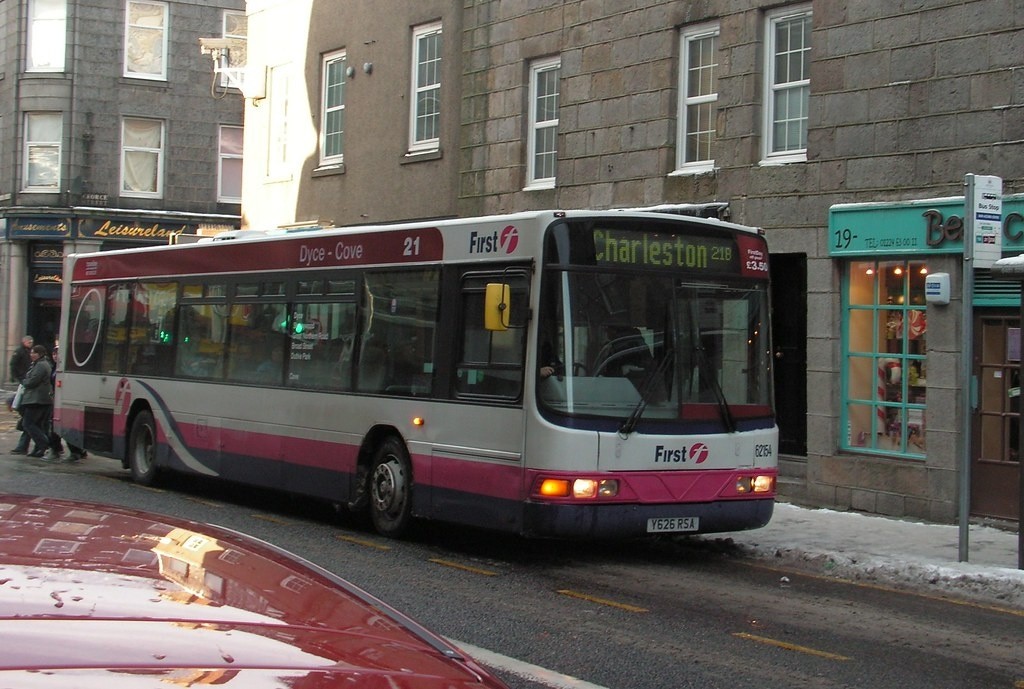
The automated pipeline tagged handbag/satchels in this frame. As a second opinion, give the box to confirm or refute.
[10,383,26,409]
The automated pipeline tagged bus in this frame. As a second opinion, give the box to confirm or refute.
[52,209,779,542]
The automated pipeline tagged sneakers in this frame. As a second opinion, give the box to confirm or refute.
[41,448,64,462]
[26,445,46,457]
[62,453,88,464]
[10,447,30,455]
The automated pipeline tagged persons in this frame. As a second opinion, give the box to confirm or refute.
[9,336,34,385]
[496,311,567,392]
[11,430,30,455]
[21,344,53,457]
[44,347,84,464]
[132,345,160,377]
[597,307,655,380]
[252,342,287,384]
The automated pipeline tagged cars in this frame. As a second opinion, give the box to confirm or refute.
[0,492,510,689]
